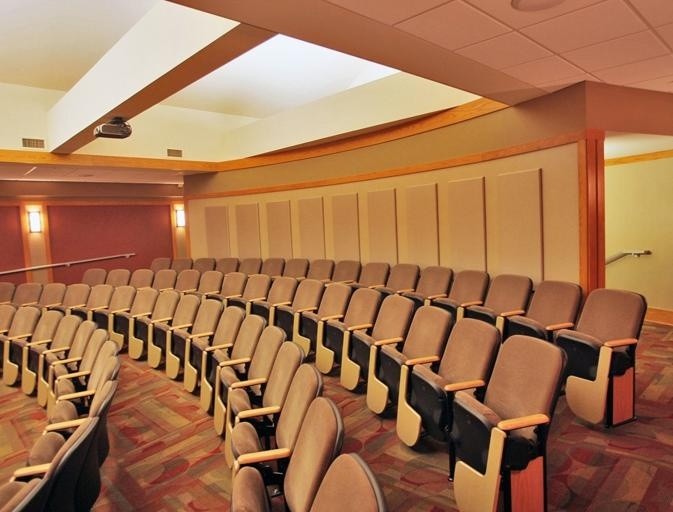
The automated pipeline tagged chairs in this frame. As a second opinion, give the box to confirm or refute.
[315,287,381,375]
[108,286,158,353]
[40,380,118,468]
[369,264,421,295]
[0,416,100,512]
[262,258,285,279]
[166,299,224,379]
[366,305,454,413]
[54,340,119,417]
[201,315,266,413]
[393,266,454,303]
[320,260,361,288]
[35,283,66,310]
[217,257,238,275]
[555,288,648,428]
[447,333,569,512]
[0,304,15,348]
[0,282,16,306]
[153,270,175,291]
[129,290,179,360]
[150,257,170,274]
[293,282,350,360]
[93,285,136,331]
[201,272,247,308]
[195,258,216,276]
[11,282,43,308]
[340,293,415,392]
[0,478,54,512]
[496,280,584,346]
[232,397,344,512]
[81,268,107,285]
[46,328,110,423]
[457,274,534,324]
[148,294,200,368]
[3,310,64,386]
[232,363,325,490]
[327,262,390,289]
[53,283,91,316]
[54,357,121,435]
[0,307,41,376]
[184,306,246,393]
[72,284,115,321]
[239,258,263,277]
[36,320,97,408]
[223,274,271,312]
[214,325,287,436]
[396,318,502,448]
[423,270,489,307]
[245,277,297,318]
[21,315,82,395]
[104,269,130,285]
[298,259,335,284]
[282,259,309,281]
[224,341,303,469]
[308,453,388,512]
[160,270,200,294]
[269,278,323,333]
[171,258,193,275]
[183,271,222,299]
[129,268,154,288]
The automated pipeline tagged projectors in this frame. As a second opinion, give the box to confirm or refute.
[94,117,132,139]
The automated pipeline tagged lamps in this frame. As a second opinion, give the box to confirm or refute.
[27,209,44,235]
[175,209,186,227]
